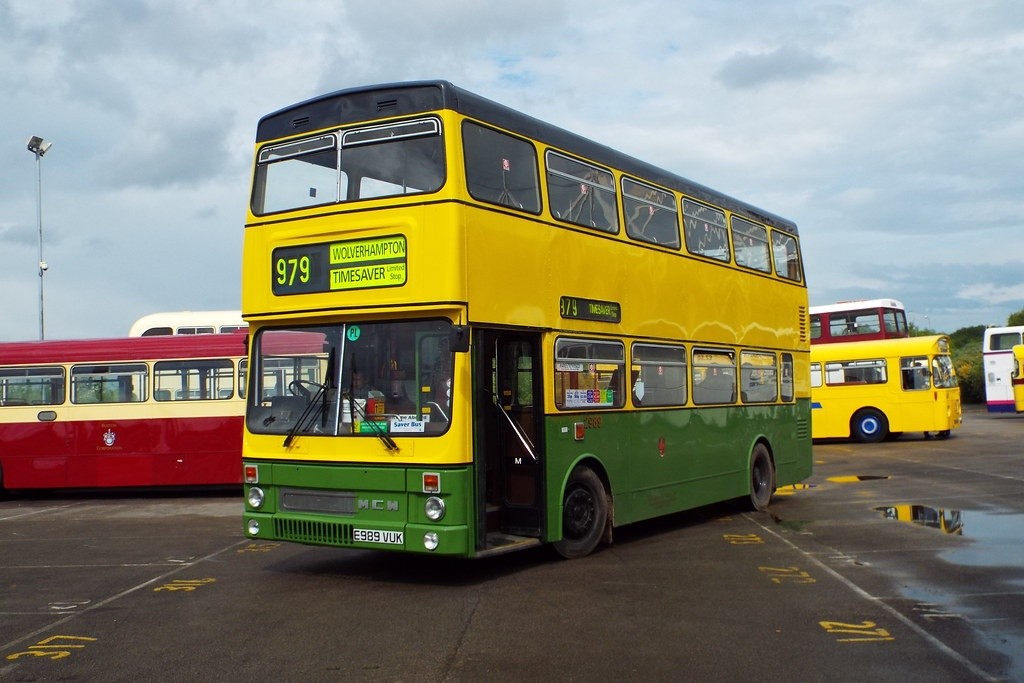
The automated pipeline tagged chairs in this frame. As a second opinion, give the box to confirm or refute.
[349,351,390,395]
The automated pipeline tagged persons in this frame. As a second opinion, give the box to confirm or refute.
[349,369,375,397]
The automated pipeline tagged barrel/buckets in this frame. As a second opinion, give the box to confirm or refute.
[342,399,366,422]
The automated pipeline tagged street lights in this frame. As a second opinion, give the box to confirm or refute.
[24,134,54,341]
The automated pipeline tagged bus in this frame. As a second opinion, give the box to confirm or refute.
[127,307,331,400]
[1010,344,1024,414]
[593,334,963,444]
[0,326,323,489]
[238,78,813,562]
[982,325,1024,418]
[810,298,910,347]
